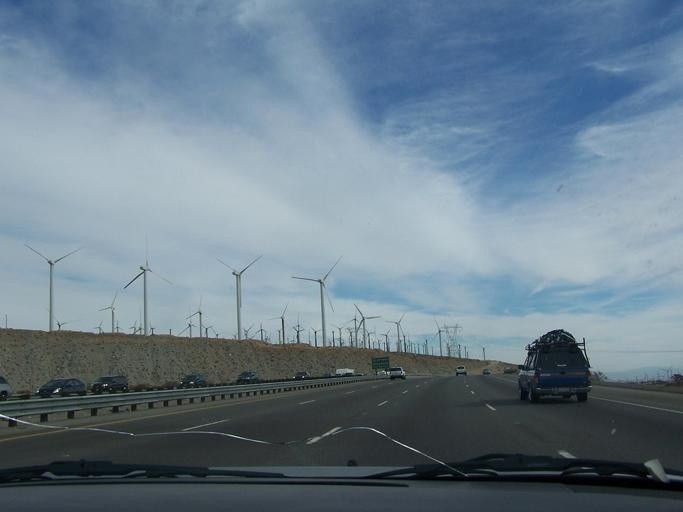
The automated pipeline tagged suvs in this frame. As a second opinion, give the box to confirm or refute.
[517,337,594,404]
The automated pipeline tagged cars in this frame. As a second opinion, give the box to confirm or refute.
[389,367,406,380]
[180,374,207,388]
[91,376,130,393]
[482,368,492,375]
[503,366,513,373]
[235,371,259,384]
[39,377,87,398]
[455,366,467,376]
[293,370,311,379]
